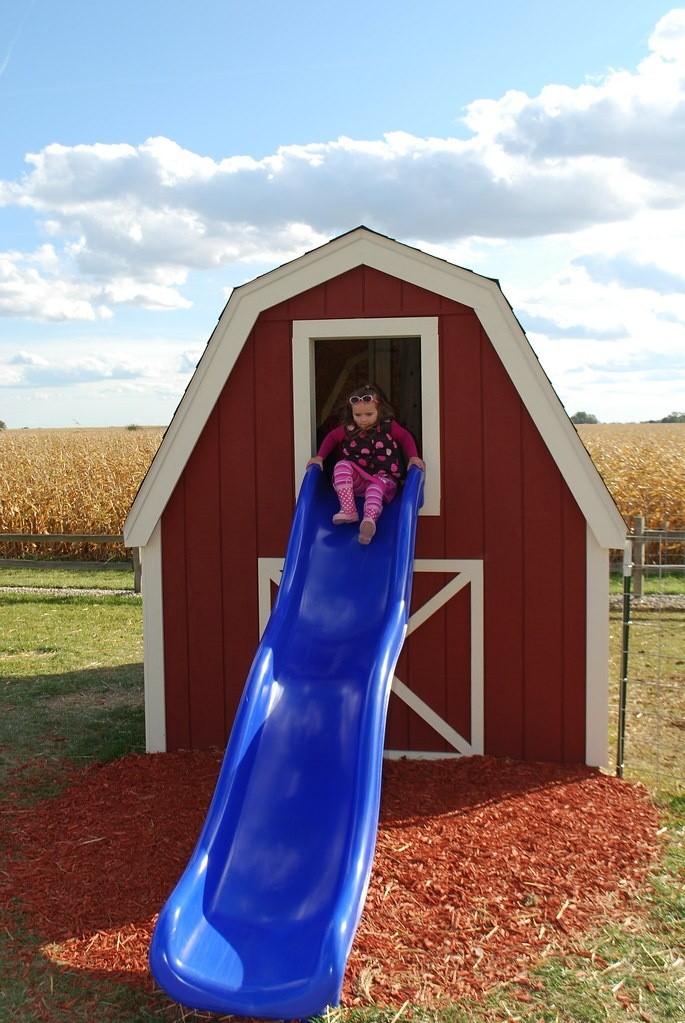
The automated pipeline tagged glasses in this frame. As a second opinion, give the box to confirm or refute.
[350,395,378,405]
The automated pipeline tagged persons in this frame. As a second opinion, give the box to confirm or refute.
[307,382,425,544]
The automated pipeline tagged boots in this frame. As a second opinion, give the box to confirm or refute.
[358,503,384,545]
[332,477,358,524]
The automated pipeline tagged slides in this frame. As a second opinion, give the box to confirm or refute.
[149,454,426,1018]
